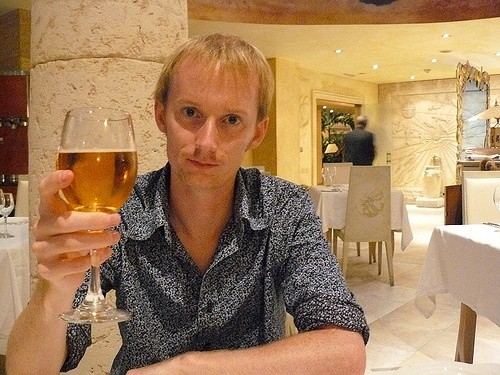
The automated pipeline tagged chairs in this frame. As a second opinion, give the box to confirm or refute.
[14,180,29,217]
[323,162,353,187]
[332,166,396,287]
[462,171,500,225]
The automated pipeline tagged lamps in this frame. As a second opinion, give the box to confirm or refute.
[468,101,500,148]
[324,143,339,163]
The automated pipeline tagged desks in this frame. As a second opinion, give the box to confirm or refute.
[0,217,29,355]
[416,224,500,363]
[307,186,413,263]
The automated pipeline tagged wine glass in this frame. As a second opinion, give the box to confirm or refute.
[492,186,500,232]
[54,105,138,325]
[0,192,15,239]
[321,166,336,187]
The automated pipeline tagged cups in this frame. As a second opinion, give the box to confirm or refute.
[0,189,6,209]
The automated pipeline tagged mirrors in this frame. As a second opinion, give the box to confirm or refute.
[455,59,492,184]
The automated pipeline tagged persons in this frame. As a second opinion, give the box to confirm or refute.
[6,34,370,375]
[344,116,377,165]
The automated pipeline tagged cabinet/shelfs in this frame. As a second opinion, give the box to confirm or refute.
[458,161,500,171]
